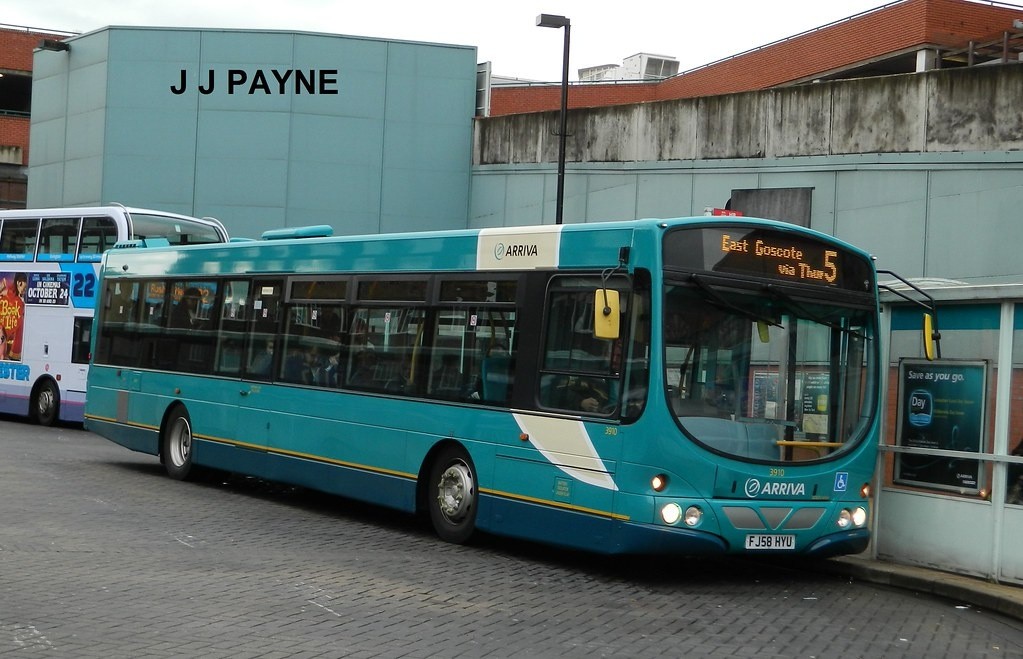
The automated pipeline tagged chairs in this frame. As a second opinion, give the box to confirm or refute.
[107,303,738,422]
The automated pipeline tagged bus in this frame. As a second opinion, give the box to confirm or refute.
[1,203,242,428]
[82,223,944,594]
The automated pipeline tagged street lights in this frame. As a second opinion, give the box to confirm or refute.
[535,12,580,227]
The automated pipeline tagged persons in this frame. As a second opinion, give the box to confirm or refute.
[551,338,639,420]
[107,279,520,406]
[4,272,26,361]
